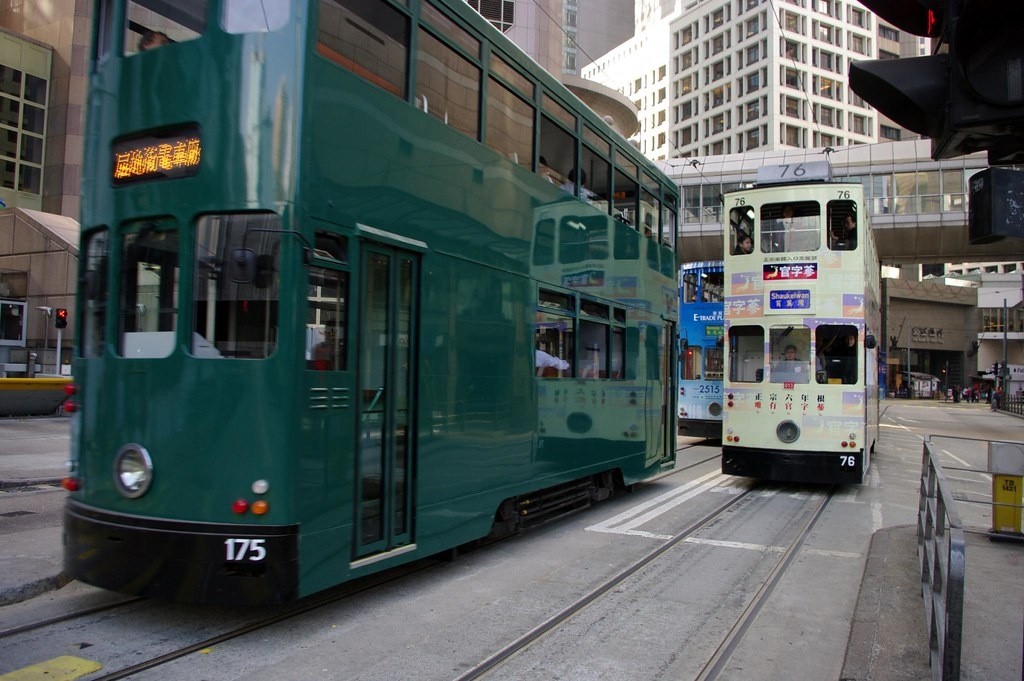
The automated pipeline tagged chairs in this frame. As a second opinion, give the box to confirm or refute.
[536,361,621,380]
[416,93,631,225]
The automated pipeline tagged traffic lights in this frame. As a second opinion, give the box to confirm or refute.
[55,308,68,328]
[993,362,998,374]
[942,367,946,374]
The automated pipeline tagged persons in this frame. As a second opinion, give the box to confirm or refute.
[734,235,753,255]
[688,291,697,303]
[645,223,652,238]
[841,334,858,383]
[540,156,553,183]
[535,349,569,371]
[138,31,168,52]
[310,319,344,370]
[952,384,1002,411]
[830,214,857,247]
[776,206,803,252]
[774,344,806,373]
[559,168,587,201]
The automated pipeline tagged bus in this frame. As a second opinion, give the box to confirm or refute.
[724,161,881,484]
[59,0,681,607]
[676,261,724,421]
